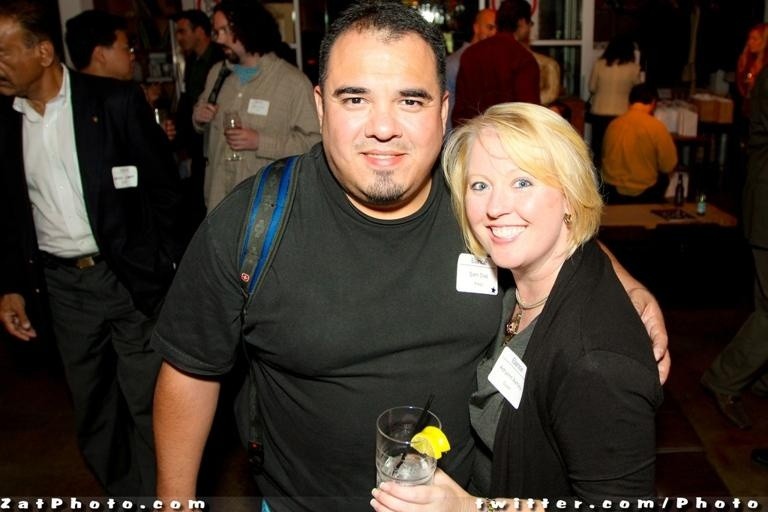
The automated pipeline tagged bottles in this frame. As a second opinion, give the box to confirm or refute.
[438,10,454,55]
[695,189,707,216]
[675,173,685,206]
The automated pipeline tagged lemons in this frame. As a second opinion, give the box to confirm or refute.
[410,425,451,460]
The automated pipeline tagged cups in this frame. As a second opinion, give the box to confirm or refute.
[153,106,167,133]
[373,404,443,495]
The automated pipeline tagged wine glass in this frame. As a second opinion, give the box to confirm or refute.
[220,110,247,162]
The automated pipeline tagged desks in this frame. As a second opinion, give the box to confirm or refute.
[601,197,736,314]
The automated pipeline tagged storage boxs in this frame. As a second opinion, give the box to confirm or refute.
[690,89,734,123]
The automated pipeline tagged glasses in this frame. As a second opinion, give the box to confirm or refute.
[210,20,234,41]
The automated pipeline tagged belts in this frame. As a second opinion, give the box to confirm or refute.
[39,252,105,269]
